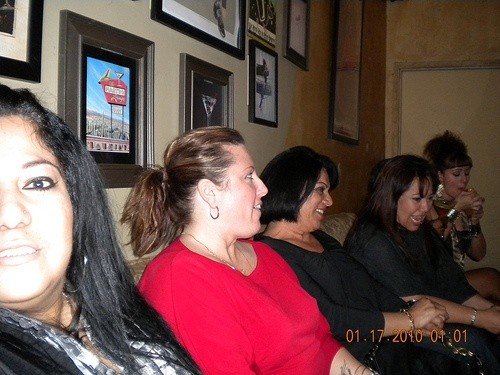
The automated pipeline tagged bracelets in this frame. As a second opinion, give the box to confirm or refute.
[469,222,481,235]
[471,308,477,325]
[446,208,460,223]
[474,233,482,240]
[413,296,416,301]
[355,365,362,374]
[362,367,366,375]
[403,309,414,331]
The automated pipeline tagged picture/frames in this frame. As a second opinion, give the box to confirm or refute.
[281,0,313,70]
[178,52,235,137]
[329,0,365,147]
[0,0,45,84]
[245,39,278,128]
[58,9,155,190]
[151,0,248,59]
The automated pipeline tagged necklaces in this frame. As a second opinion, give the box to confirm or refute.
[437,187,472,242]
[64,295,73,314]
[182,232,245,274]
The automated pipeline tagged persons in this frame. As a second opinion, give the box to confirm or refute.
[0,84,202,375]
[121,126,377,375]
[421,131,500,299]
[344,153,500,375]
[252,145,469,375]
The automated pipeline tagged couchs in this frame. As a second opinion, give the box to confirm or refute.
[123,212,359,294]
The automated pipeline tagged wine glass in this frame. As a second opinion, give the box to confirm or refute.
[464,189,478,238]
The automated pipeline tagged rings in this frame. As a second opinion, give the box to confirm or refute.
[481,211,483,214]
